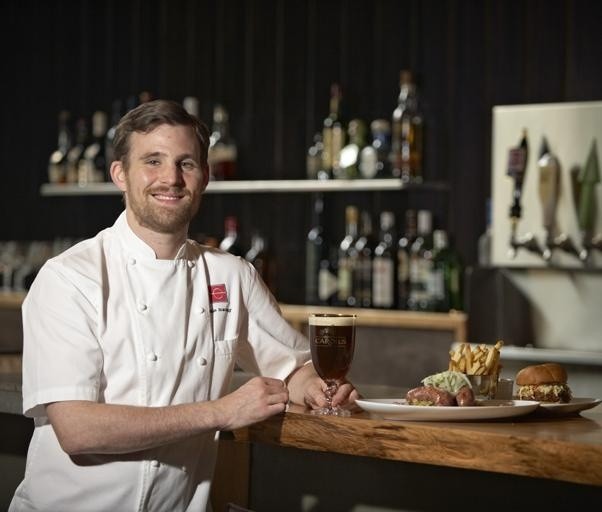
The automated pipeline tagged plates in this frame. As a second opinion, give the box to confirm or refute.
[356,396,540,421]
[538,396,602,417]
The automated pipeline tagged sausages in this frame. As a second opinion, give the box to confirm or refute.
[406,386,454,406]
[455,385,474,406]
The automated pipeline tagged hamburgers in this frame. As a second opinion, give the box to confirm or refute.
[516,362,572,405]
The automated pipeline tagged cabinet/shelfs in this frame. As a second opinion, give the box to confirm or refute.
[2,172,470,393]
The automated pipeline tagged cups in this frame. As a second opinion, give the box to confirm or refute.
[495,378,513,399]
[464,373,500,401]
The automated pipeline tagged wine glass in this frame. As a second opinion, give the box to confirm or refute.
[308,312,357,415]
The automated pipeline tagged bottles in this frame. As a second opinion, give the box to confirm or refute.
[45,86,236,190]
[1,214,271,298]
[303,70,424,183]
[318,204,463,315]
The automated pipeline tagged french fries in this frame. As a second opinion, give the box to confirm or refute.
[448,339,505,376]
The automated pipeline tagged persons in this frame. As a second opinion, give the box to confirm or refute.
[8,100,367,512]
